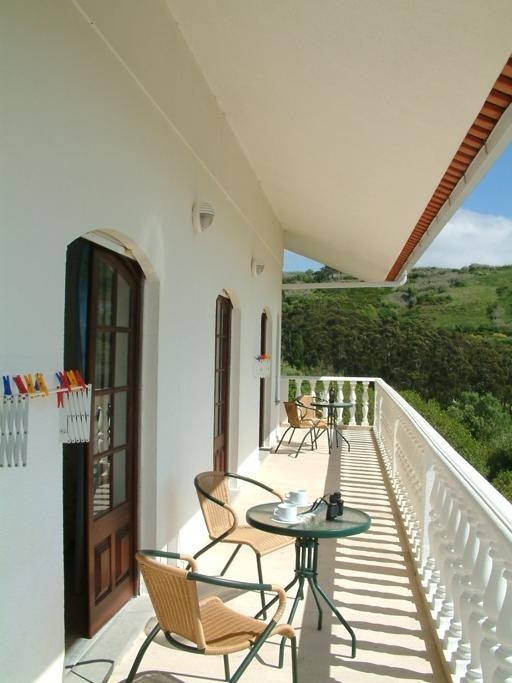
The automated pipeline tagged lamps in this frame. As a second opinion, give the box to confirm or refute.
[192,199,216,234]
[250,255,265,280]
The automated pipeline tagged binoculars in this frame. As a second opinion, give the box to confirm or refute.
[327,492,343,520]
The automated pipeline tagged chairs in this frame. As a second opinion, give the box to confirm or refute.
[275,401,331,458]
[125,550,298,683]
[184,470,304,620]
[287,394,340,451]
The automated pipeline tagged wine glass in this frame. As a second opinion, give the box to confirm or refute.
[320,392,329,404]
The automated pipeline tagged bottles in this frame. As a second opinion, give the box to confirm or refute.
[330,382,334,403]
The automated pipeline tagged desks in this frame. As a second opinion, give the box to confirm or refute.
[311,402,352,454]
[245,501,371,669]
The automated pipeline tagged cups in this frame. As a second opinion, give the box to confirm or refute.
[273,502,298,521]
[286,489,307,506]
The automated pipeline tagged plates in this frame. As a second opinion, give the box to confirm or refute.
[298,500,312,508]
[270,516,304,524]
